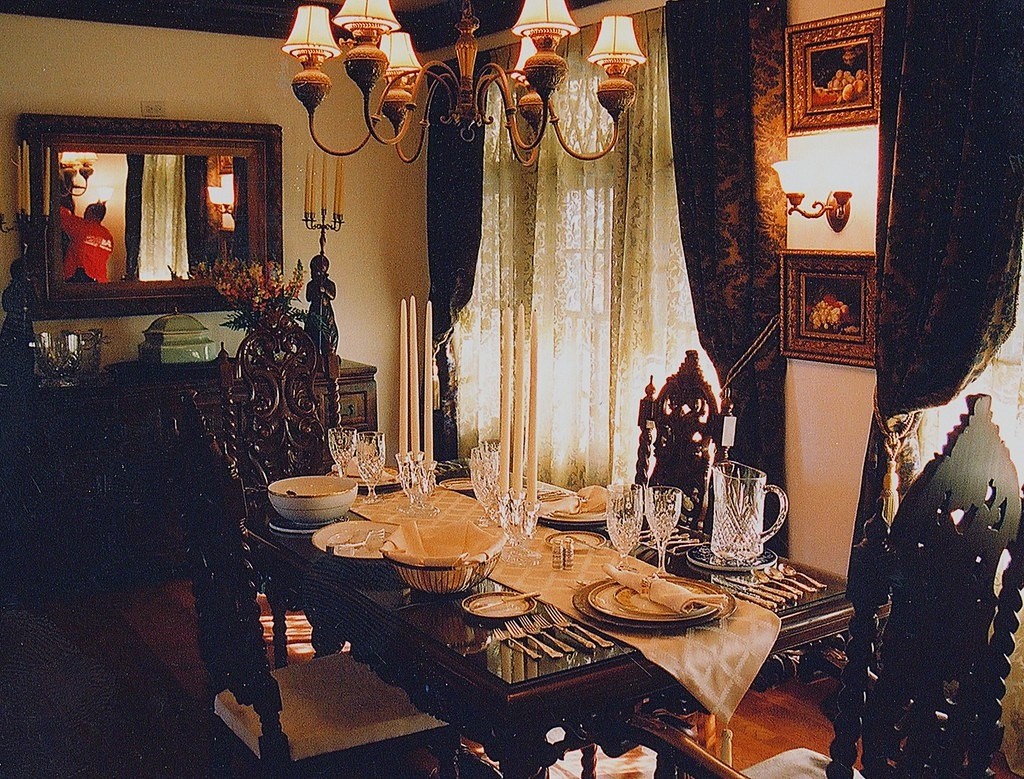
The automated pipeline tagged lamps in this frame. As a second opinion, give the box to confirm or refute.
[771,160,852,235]
[56,151,97,198]
[207,162,237,221]
[283,2,647,169]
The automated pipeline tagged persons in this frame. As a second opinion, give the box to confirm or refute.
[58,202,114,284]
[306,255,339,354]
[0,257,46,386]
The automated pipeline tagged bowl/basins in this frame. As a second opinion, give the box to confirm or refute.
[268,476,359,525]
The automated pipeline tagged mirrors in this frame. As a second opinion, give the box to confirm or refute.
[16,110,283,320]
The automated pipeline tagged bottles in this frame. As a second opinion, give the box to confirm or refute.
[550,539,575,569]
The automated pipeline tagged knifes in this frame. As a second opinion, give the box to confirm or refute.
[700,576,799,608]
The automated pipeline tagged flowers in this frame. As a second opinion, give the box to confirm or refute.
[212,266,305,325]
[167,257,246,283]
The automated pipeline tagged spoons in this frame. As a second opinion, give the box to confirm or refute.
[747,563,827,597]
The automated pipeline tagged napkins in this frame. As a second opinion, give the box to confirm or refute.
[603,560,726,619]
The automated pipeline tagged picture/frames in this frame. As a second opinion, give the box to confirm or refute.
[782,8,883,137]
[772,251,879,370]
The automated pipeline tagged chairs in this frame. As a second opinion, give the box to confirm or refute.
[625,351,721,560]
[172,401,462,779]
[641,396,1024,779]
[216,318,347,484]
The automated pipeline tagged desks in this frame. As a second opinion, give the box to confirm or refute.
[238,465,889,779]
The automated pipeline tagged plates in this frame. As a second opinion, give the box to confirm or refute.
[440,477,614,551]
[461,591,536,618]
[312,520,407,559]
[572,576,736,629]
[328,464,402,486]
[269,516,350,535]
[687,539,778,573]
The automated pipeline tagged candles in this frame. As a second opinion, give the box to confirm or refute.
[334,157,345,217]
[399,299,408,461]
[320,151,329,218]
[422,301,434,465]
[496,299,511,504]
[527,308,540,507]
[513,300,524,502]
[304,149,316,214]
[409,296,419,458]
[41,148,51,216]
[14,142,31,220]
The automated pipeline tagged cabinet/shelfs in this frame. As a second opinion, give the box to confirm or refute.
[0,357,379,486]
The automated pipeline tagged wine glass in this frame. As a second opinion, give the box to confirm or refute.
[468,442,544,561]
[393,451,438,516]
[356,431,386,505]
[602,483,683,580]
[328,427,357,480]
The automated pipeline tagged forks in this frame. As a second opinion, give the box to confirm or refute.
[518,615,576,653]
[493,628,542,660]
[532,613,596,649]
[504,620,564,659]
[543,603,614,648]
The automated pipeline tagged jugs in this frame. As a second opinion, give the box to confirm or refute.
[709,459,789,560]
